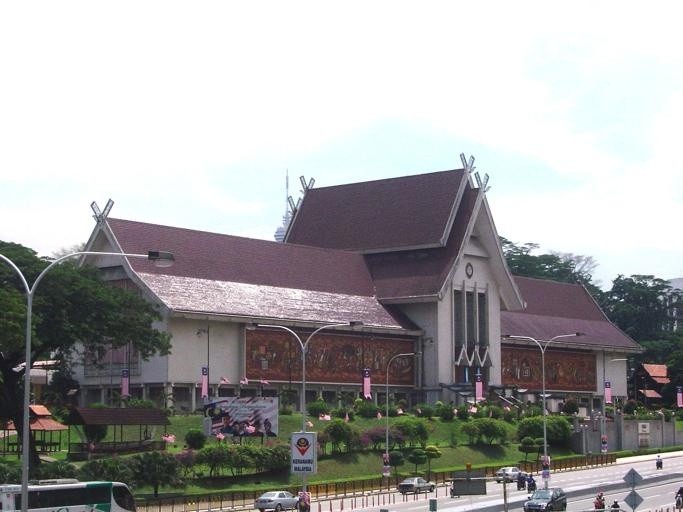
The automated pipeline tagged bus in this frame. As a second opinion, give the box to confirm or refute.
[0,479,136,512]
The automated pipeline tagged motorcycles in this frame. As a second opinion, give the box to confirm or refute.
[517,476,536,493]
[675,492,682,508]
[595,497,604,509]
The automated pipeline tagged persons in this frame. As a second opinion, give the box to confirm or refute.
[517,471,536,493]
[219,412,233,433]
[299,493,310,512]
[593,492,620,512]
[231,421,277,436]
[656,455,662,466]
[674,487,683,503]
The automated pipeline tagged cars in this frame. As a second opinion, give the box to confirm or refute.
[398,477,436,495]
[254,490,299,511]
[524,487,565,512]
[495,467,529,483]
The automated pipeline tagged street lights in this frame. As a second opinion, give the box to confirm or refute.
[603,359,629,454]
[501,331,584,490]
[254,322,363,492]
[0,250,173,512]
[384,352,422,491]
